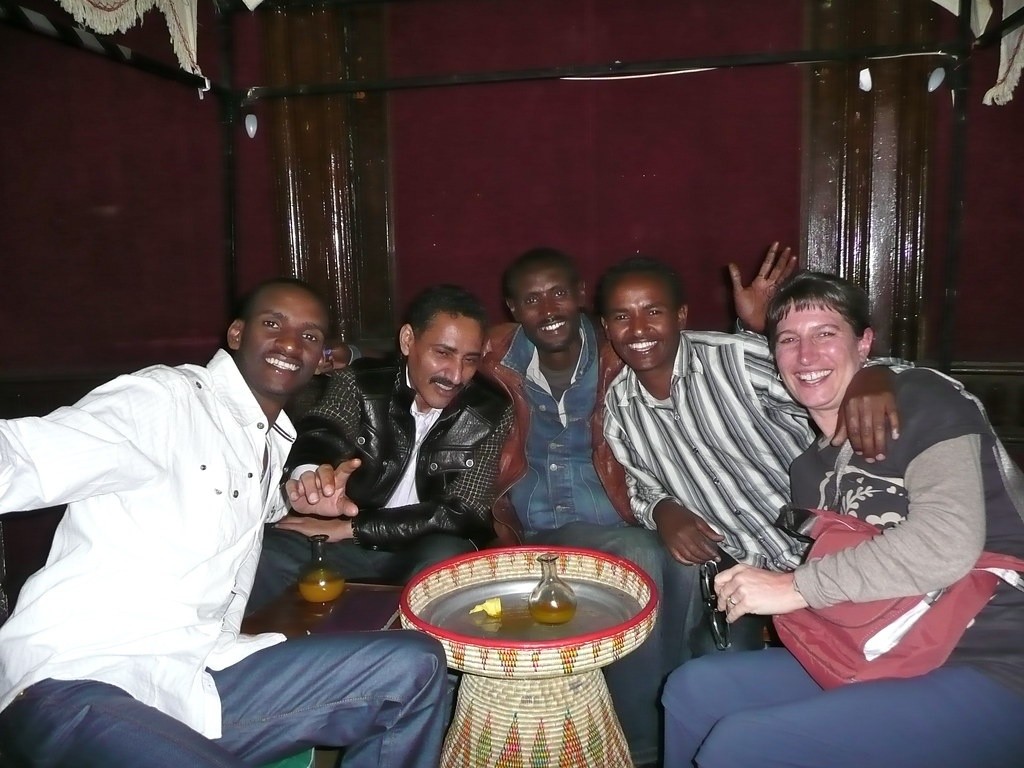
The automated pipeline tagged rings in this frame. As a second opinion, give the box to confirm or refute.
[729,599,735,606]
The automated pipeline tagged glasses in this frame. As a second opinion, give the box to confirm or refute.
[700,560,732,651]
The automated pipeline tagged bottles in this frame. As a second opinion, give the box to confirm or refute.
[529,555,576,624]
[297,534,345,603]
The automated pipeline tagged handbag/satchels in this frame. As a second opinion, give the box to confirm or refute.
[772,505,1024,690]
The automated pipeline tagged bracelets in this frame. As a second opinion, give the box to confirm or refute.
[346,344,361,366]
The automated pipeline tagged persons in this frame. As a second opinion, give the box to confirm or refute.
[596,261,915,657]
[1,278,448,768]
[245,282,515,616]
[662,272,1024,768]
[318,240,798,768]
